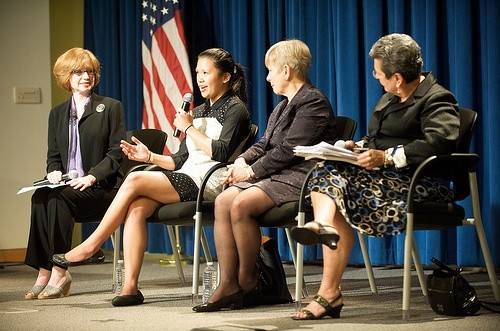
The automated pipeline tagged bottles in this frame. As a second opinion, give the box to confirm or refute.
[114,260,125,296]
[203,262,217,304]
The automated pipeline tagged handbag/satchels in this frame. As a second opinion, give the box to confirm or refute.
[426,258,481,316]
[235,235,294,309]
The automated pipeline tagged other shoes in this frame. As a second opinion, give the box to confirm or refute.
[111,289,144,307]
[52,249,105,267]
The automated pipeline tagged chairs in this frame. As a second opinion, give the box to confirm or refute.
[358,107,500,322]
[71,129,186,294]
[144,124,259,306]
[222,116,378,298]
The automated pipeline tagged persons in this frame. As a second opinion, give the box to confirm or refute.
[24,47,126,300]
[290,33,461,321]
[53,48,252,306]
[193,40,336,313]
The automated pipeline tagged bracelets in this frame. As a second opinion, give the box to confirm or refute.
[247,166,255,181]
[384,150,389,168]
[145,151,154,163]
[185,124,194,135]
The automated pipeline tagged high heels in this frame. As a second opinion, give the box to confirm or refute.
[192,288,244,312]
[38,271,72,300]
[25,284,46,299]
[292,293,344,320]
[236,282,259,309]
[290,221,340,250]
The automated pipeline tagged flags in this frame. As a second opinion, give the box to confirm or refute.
[142,0,194,156]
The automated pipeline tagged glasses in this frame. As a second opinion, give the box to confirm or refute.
[73,68,97,76]
[373,70,390,80]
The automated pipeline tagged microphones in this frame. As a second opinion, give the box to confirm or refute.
[335,139,347,149]
[33,170,78,186]
[173,93,193,137]
